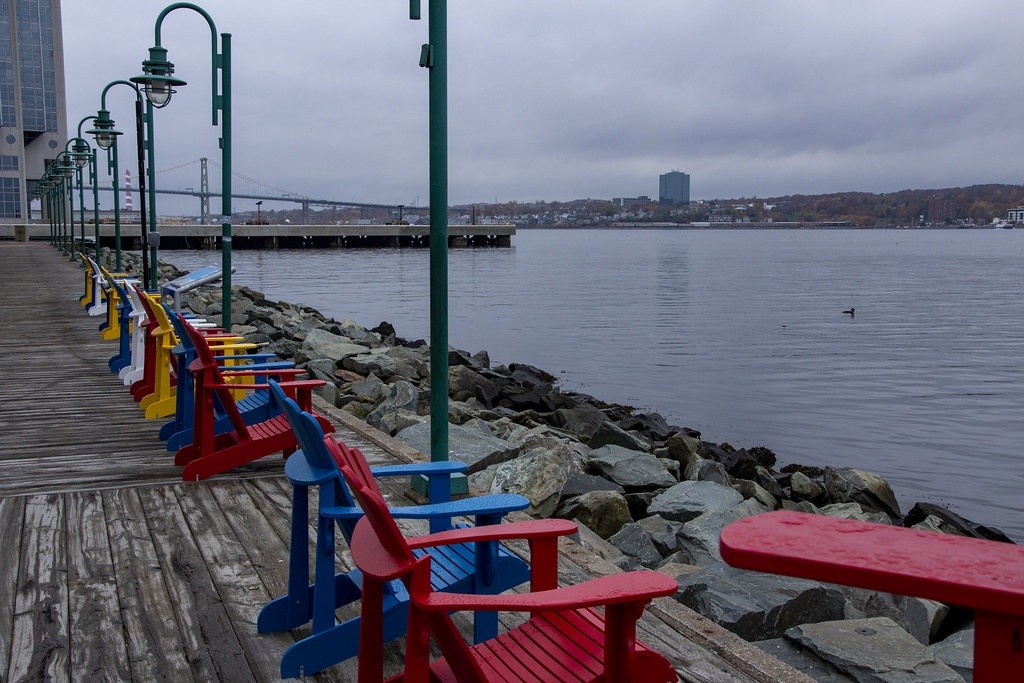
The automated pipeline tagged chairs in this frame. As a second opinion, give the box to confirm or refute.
[258,382,680,683]
[76,254,334,484]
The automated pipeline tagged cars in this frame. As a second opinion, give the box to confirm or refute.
[402,215,428,225]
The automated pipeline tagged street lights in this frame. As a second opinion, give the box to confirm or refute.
[256,201,262,224]
[398,205,404,225]
[130,2,232,330]
[34,116,122,274]
[472,206,475,225]
[85,80,159,292]
